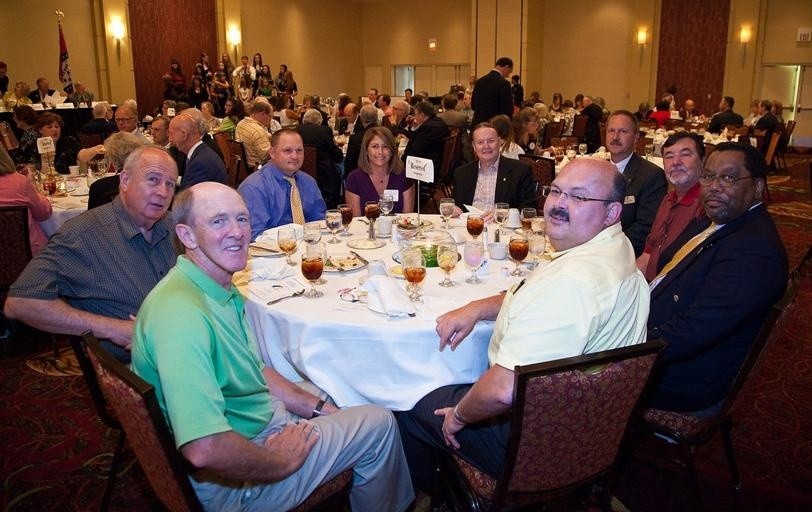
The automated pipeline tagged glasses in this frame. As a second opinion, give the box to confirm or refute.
[697,172,760,189]
[541,185,615,207]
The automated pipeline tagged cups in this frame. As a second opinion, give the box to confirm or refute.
[69,166,80,176]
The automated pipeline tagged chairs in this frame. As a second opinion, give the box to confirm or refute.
[59,297,127,512]
[1,92,346,349]
[340,94,796,245]
[441,342,667,511]
[79,328,356,512]
[622,248,795,512]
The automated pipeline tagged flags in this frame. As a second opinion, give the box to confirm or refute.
[58,27,73,97]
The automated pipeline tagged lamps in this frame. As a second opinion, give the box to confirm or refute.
[740,30,751,43]
[638,31,647,44]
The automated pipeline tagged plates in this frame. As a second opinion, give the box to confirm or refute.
[55,180,89,209]
[249,243,297,256]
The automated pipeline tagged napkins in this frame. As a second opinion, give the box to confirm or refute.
[231,258,295,285]
[363,275,416,319]
[255,223,306,249]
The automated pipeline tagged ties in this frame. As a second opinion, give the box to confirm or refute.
[656,222,716,282]
[282,173,306,226]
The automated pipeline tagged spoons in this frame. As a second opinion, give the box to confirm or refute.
[266,289,304,305]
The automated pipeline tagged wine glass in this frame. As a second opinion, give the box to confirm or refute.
[277,195,546,297]
[43,181,57,204]
[87,159,107,178]
[549,142,589,166]
[644,144,653,161]
